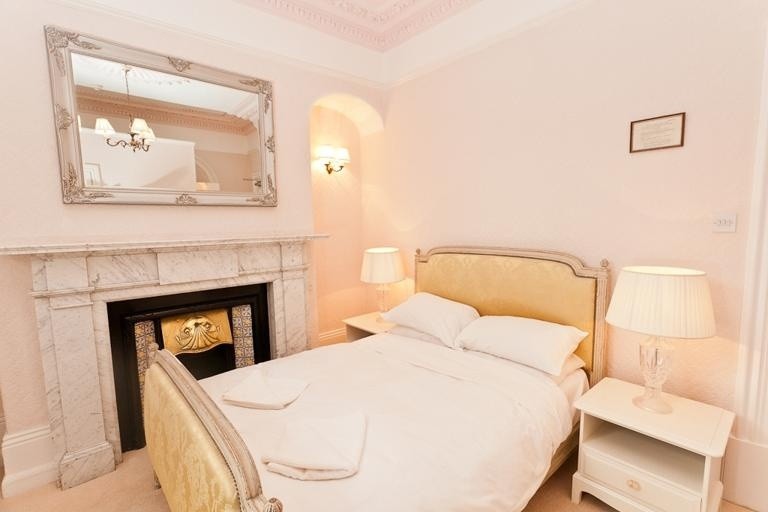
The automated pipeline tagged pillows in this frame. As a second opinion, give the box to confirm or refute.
[380,292,590,387]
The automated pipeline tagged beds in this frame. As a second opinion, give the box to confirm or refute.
[139,244,609,512]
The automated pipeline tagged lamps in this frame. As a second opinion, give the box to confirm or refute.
[361,247,407,323]
[92,63,156,153]
[317,142,350,174]
[605,264,717,415]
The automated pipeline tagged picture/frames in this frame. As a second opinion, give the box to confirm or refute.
[629,112,686,153]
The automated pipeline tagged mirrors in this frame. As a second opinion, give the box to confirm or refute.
[45,23,278,207]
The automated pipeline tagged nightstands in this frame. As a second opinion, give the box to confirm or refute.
[341,312,392,343]
[569,375,736,512]
